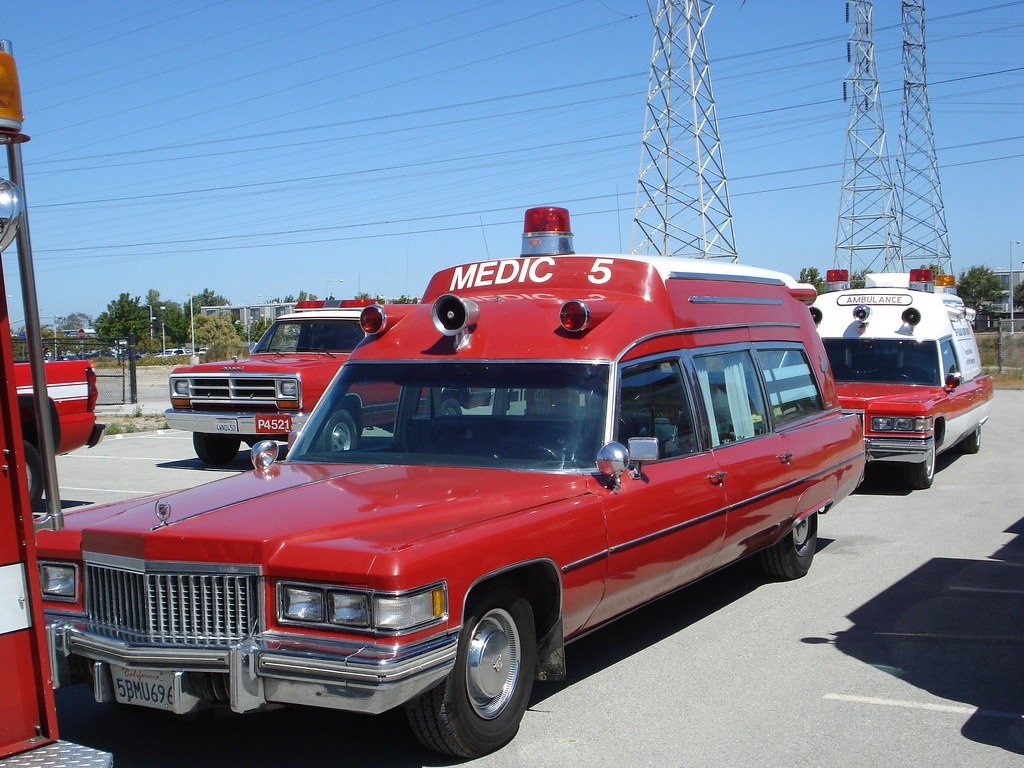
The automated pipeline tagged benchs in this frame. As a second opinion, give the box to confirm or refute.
[402,407,654,460]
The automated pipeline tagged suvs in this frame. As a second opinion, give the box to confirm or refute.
[776,269,993,490]
[26,206,865,761]
[164,301,526,464]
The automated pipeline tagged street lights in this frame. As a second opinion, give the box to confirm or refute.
[325,280,344,299]
[1010,240,1021,332]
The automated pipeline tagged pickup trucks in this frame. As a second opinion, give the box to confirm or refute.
[13,359,107,513]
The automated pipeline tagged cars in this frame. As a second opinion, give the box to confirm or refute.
[152,347,208,358]
[55,348,140,361]
